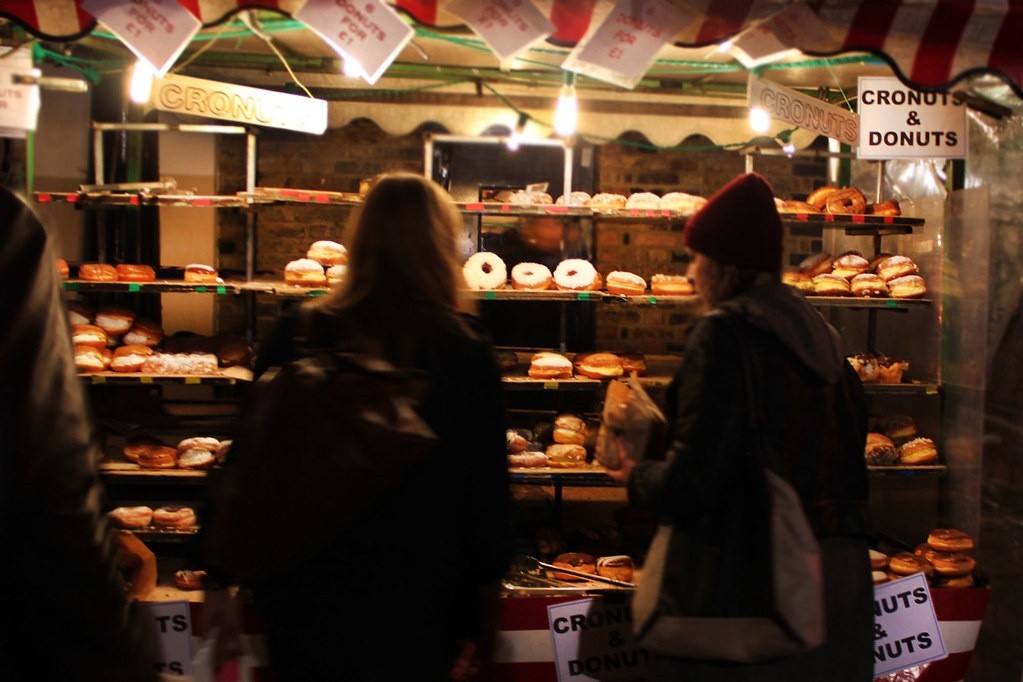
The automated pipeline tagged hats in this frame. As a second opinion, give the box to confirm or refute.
[680,173,785,275]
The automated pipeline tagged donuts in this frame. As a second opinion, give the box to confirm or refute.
[53,240,348,588]
[460,184,976,590]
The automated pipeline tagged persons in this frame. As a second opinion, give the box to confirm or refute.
[200,173,511,682]
[0,176,170,682]
[916,292,1022,682]
[626,169,877,682]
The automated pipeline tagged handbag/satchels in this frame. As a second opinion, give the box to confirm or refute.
[632,465,821,655]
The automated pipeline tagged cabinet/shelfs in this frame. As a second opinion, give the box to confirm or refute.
[31,190,948,549]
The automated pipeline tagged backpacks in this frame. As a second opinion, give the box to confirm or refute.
[197,346,444,650]
